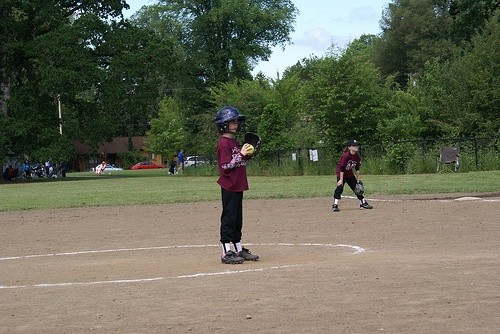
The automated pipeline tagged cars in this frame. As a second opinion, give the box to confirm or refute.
[130,161,167,170]
[92,163,123,172]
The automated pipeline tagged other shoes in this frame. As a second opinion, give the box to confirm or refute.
[360,202,373,209]
[331,204,340,212]
[236,247,259,261]
[221,250,244,264]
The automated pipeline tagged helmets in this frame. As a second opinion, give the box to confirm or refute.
[214,107,246,124]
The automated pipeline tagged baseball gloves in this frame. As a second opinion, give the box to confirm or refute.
[354,179,364,196]
[242,133,262,160]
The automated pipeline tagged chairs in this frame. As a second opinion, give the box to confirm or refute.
[436,146,460,174]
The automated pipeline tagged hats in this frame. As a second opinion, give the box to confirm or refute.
[347,140,360,146]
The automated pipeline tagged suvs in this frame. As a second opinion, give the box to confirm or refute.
[180,156,213,167]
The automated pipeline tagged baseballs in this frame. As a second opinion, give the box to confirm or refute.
[245,144,255,156]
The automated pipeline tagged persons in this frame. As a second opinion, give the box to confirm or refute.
[332,139,373,212]
[215,106,259,264]
[168,149,186,175]
[97,161,106,174]
[4,158,66,182]
